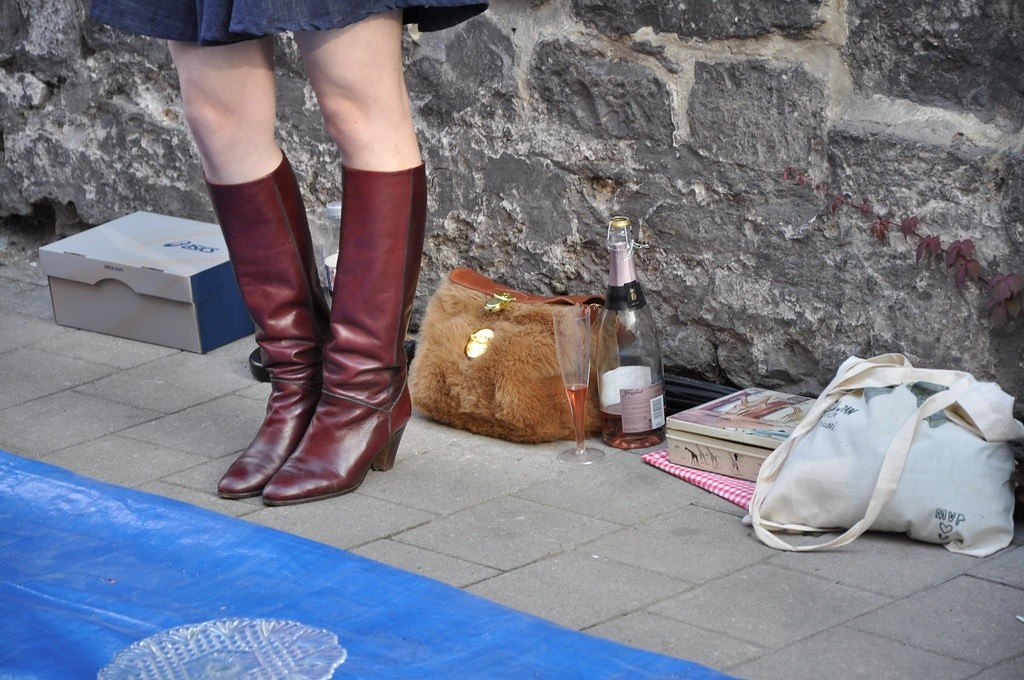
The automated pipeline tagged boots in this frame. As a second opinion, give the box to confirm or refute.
[257,161,429,500]
[192,147,335,505]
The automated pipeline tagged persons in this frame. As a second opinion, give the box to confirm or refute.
[89,0,489,508]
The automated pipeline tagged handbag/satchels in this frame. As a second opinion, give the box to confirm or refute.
[411,261,612,444]
[733,347,1023,565]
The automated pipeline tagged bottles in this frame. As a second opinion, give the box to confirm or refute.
[597,217,668,450]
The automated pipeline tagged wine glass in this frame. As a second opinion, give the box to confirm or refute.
[556,304,607,464]
